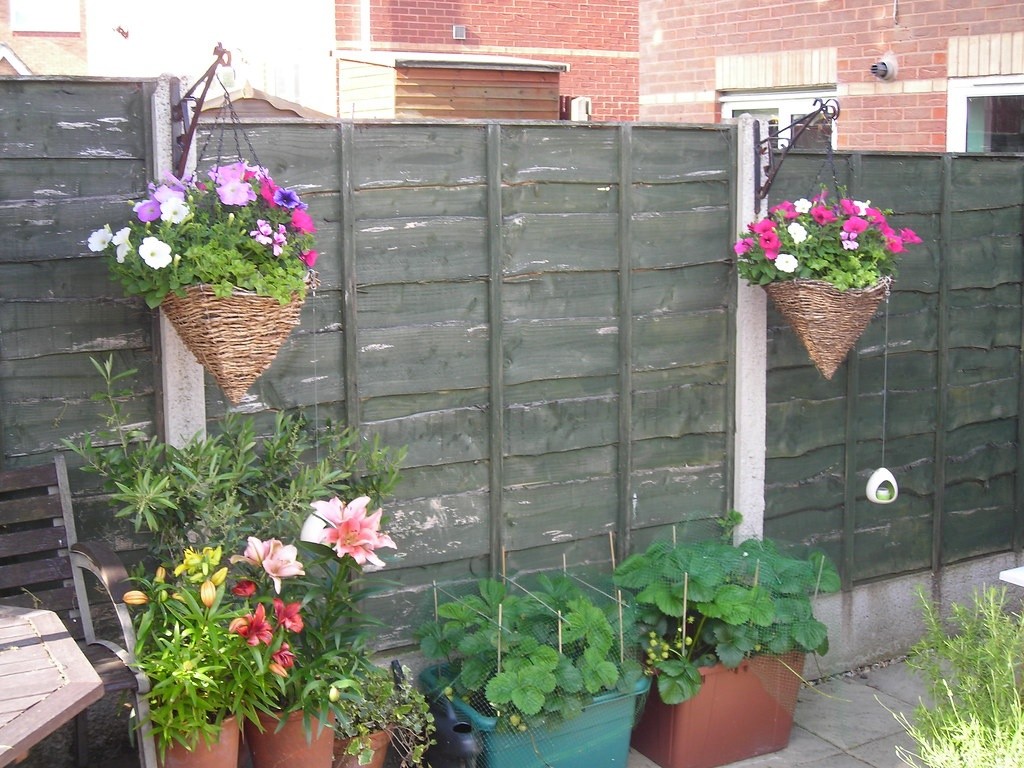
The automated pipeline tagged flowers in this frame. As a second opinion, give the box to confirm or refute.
[62,352,410,742]
[734,189,922,284]
[87,161,317,307]
[115,545,306,768]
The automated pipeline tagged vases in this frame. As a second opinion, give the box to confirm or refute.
[332,722,394,768]
[152,710,239,768]
[244,706,336,768]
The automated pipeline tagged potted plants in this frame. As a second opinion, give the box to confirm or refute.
[411,572,650,768]
[610,511,842,768]
